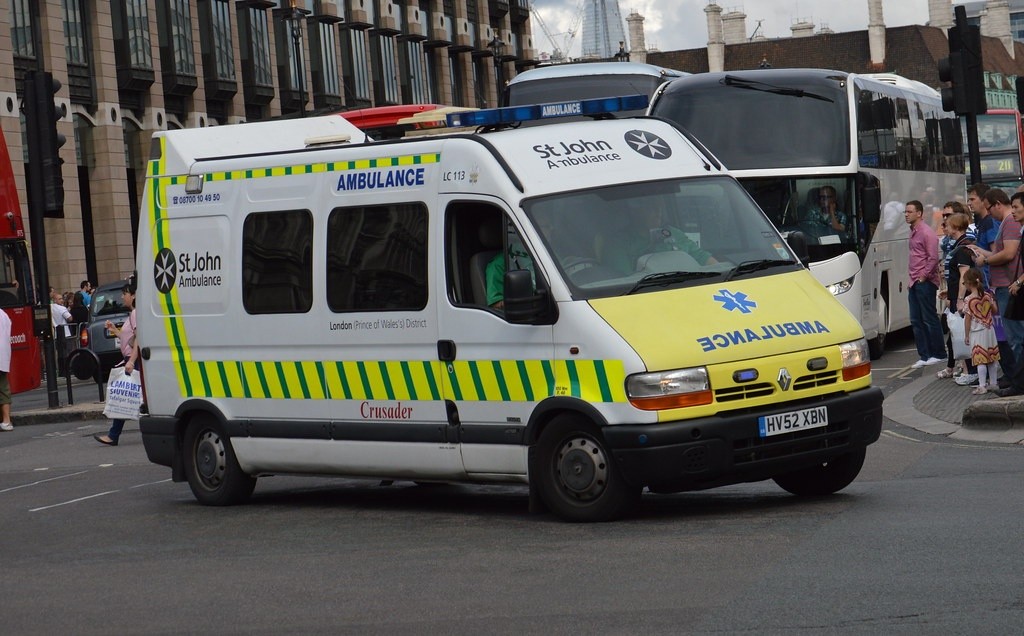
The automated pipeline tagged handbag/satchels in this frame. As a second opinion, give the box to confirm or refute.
[103,367,143,420]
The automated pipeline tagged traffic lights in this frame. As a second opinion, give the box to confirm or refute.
[35,70,66,159]
[938,24,988,115]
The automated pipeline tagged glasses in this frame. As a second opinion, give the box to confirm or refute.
[942,213,954,218]
[941,223,947,229]
[986,201,998,211]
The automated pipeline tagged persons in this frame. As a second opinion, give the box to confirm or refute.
[905,184,1024,397]
[800,186,847,242]
[881,187,964,257]
[94,284,147,447]
[606,194,718,275]
[48,281,95,371]
[0,309,13,431]
[486,212,554,310]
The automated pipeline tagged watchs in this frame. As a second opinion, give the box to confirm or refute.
[1015,280,1022,286]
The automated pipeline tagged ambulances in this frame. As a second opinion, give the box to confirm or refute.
[125,88,886,523]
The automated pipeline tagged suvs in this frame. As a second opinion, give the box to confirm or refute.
[80,279,140,384]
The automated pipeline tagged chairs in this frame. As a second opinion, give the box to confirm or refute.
[468,248,496,308]
[798,188,821,222]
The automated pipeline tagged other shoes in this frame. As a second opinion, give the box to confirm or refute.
[0,422,13,430]
[93,434,118,446]
[937,368,953,379]
[972,386,987,395]
[911,360,926,369]
[925,357,942,365]
[955,373,978,384]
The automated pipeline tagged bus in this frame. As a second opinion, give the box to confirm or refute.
[958,106,1024,205]
[497,57,695,129]
[638,65,968,360]
[0,117,46,405]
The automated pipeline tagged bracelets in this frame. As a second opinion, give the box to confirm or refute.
[918,279,921,283]
[983,258,988,265]
[958,297,964,300]
